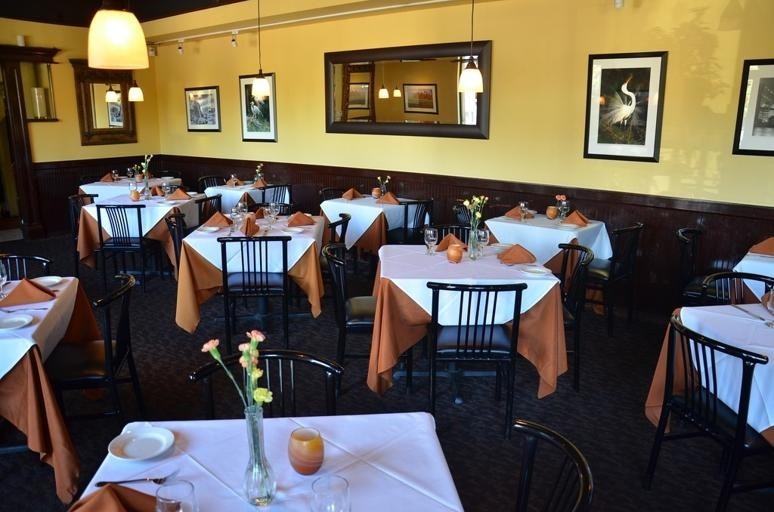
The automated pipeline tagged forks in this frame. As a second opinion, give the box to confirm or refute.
[96,472,182,488]
[727,299,774,333]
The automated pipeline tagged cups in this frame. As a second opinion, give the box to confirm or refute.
[287,425,324,478]
[311,475,351,512]
[228,198,292,244]
[150,479,197,512]
[446,242,465,263]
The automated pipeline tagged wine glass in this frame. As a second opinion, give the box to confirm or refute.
[111,168,179,215]
[1,261,9,307]
[517,201,529,224]
[423,228,438,257]
[558,200,571,222]
[474,230,490,255]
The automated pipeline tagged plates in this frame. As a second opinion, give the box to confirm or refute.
[0,315,34,331]
[107,425,177,460]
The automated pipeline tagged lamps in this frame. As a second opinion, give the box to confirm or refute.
[177,39,185,55]
[458,0,484,94]
[87,0,151,69]
[377,64,389,98]
[128,72,144,101]
[105,83,118,103]
[392,85,402,98]
[251,0,271,97]
[231,31,238,48]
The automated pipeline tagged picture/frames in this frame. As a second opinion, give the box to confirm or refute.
[729,58,774,156]
[105,90,124,128]
[348,82,369,110]
[184,87,221,132]
[238,72,278,143]
[582,50,669,163]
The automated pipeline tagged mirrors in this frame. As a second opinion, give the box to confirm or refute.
[68,57,138,147]
[323,38,494,140]
[19,61,59,123]
